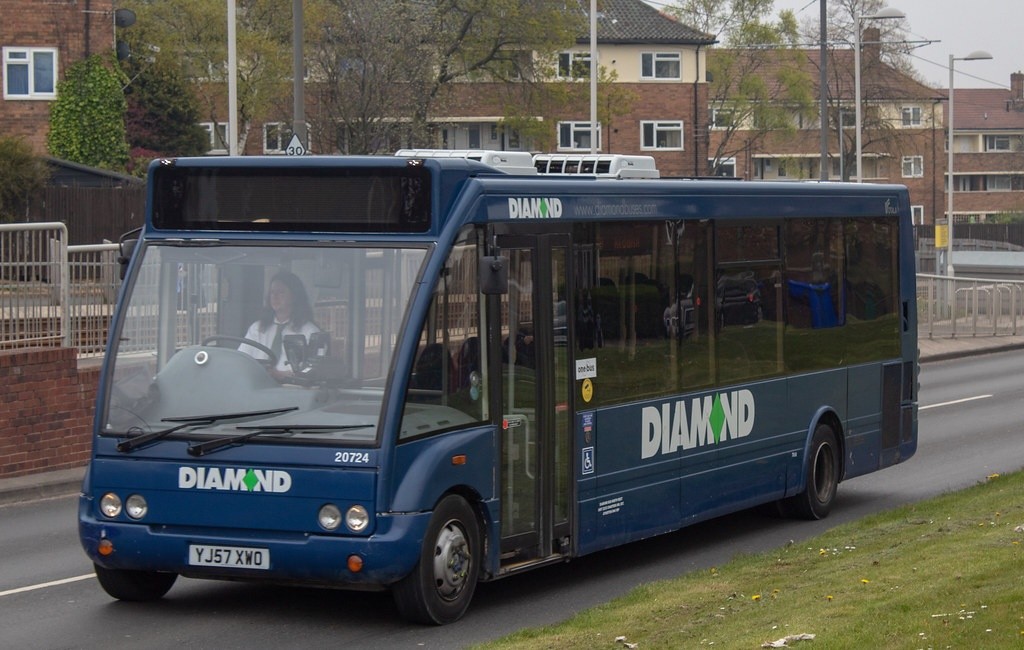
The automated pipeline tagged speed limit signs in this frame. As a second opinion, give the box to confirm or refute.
[285,133,307,156]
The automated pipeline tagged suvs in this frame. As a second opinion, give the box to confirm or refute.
[663,274,765,340]
[552,284,605,352]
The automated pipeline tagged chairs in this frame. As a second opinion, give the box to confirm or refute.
[417,270,761,392]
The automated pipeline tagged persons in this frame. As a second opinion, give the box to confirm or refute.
[835,256,888,322]
[233,270,330,390]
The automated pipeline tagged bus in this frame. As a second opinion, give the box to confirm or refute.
[77,148,923,626]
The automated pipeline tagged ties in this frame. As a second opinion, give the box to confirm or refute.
[270,323,288,361]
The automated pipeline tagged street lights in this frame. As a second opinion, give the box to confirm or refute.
[945,49,994,320]
[853,7,908,183]
